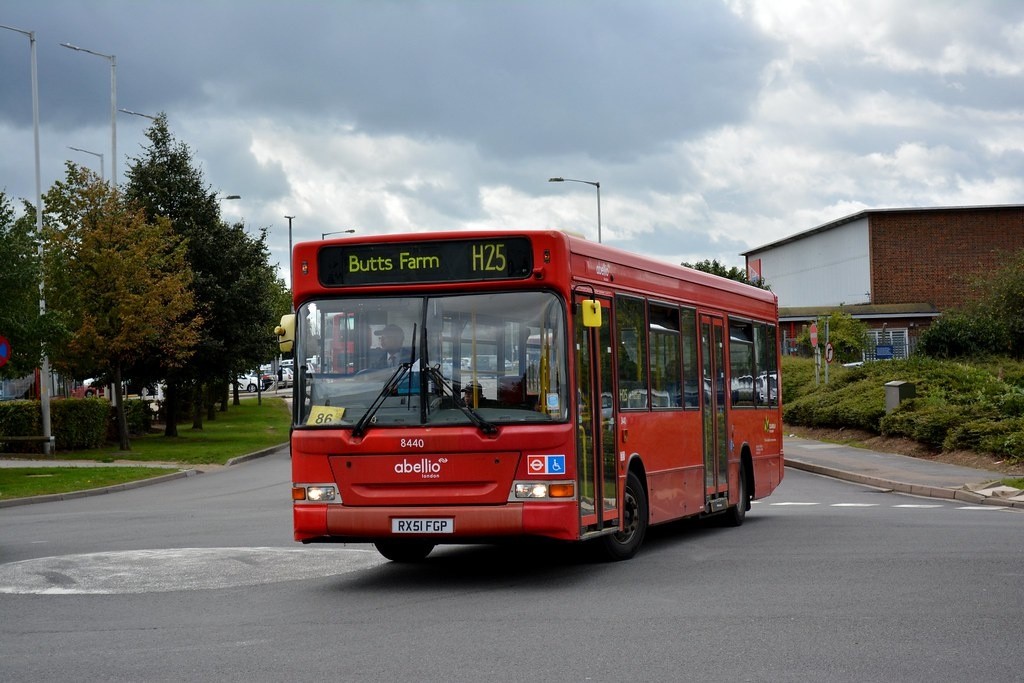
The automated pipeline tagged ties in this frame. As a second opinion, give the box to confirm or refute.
[389,356,396,368]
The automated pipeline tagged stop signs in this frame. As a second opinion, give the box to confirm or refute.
[810,324,818,348]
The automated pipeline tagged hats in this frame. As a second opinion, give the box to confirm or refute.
[374,324,405,339]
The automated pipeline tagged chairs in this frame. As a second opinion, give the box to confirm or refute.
[628,360,752,407]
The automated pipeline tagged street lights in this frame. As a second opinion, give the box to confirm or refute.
[284,215,297,313]
[321,229,355,240]
[59,41,120,191]
[68,145,107,185]
[548,178,602,242]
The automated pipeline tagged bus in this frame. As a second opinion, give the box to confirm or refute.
[272,228,788,564]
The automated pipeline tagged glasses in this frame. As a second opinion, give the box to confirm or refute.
[379,335,401,342]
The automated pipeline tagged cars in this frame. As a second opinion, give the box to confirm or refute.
[71,355,322,400]
[596,371,780,420]
[441,353,515,380]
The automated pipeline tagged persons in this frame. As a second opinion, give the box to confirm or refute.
[369,324,411,371]
[460,381,489,408]
